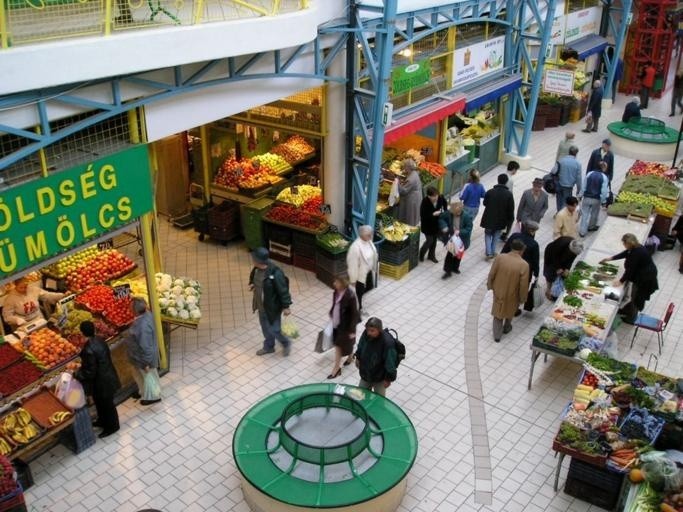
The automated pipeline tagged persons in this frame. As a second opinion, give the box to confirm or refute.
[579,78,605,133]
[124,296,163,406]
[639,59,656,110]
[623,96,642,124]
[1,276,72,333]
[355,316,398,399]
[672,214,683,276]
[603,46,624,105]
[245,245,294,357]
[71,320,120,439]
[669,69,683,117]
[325,275,362,380]
[600,232,661,325]
[345,223,379,310]
[394,132,614,343]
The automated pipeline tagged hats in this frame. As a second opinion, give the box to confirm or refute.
[531,177,546,187]
[250,246,272,265]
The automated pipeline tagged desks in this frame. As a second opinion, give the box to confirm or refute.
[528,212,658,392]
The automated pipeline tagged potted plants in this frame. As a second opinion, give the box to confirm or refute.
[525,95,574,131]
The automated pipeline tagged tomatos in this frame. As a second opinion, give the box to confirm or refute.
[75,285,134,325]
[582,371,596,387]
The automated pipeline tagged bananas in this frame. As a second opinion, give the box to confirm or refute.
[384,220,407,241]
[0,407,71,456]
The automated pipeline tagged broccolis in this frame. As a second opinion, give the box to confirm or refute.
[155,272,202,320]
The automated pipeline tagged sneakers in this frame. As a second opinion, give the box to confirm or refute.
[578,230,586,238]
[426,254,439,264]
[281,340,292,356]
[502,324,513,334]
[419,252,425,263]
[440,272,452,280]
[451,267,462,275]
[97,423,121,439]
[255,346,276,356]
[580,127,598,133]
[513,308,522,318]
[92,418,106,428]
[139,396,163,406]
[493,337,501,343]
[587,224,601,232]
[132,391,141,399]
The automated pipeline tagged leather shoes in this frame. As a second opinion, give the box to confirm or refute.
[343,358,353,366]
[326,366,343,379]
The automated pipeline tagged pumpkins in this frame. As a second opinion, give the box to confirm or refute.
[630,469,643,482]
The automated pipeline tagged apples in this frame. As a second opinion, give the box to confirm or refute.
[41,244,136,289]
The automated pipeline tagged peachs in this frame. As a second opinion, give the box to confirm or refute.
[20,327,79,368]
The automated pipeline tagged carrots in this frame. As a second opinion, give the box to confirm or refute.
[609,448,635,465]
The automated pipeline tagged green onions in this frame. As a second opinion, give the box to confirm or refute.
[321,232,348,248]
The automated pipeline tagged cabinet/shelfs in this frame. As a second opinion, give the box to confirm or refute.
[210,151,441,238]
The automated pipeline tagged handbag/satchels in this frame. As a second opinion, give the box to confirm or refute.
[523,275,543,312]
[314,318,334,354]
[445,232,465,260]
[437,224,454,246]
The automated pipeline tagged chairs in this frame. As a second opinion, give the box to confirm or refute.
[630,302,674,356]
